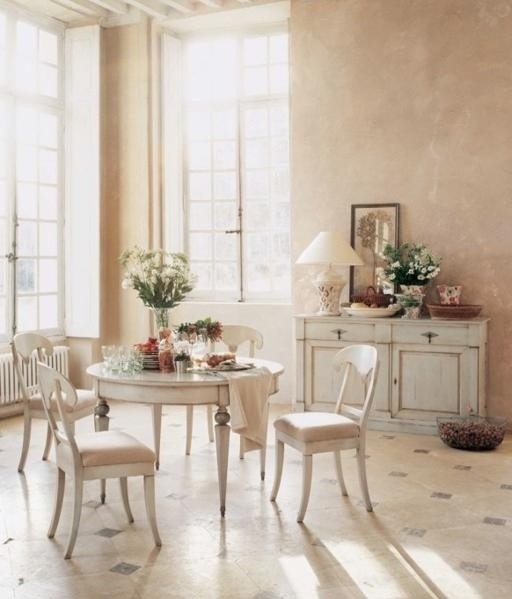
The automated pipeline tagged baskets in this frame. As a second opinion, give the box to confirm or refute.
[426,304,483,319]
[351,285,395,308]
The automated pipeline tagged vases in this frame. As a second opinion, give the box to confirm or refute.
[186,337,212,372]
[149,305,169,338]
[137,353,159,370]
[401,284,428,298]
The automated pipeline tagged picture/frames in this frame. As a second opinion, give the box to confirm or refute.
[348,200,401,302]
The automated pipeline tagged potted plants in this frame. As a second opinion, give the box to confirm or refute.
[397,296,422,320]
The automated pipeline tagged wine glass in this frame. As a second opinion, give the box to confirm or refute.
[174,328,208,372]
[98,343,143,377]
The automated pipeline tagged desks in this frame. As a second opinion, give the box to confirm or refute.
[81,353,287,520]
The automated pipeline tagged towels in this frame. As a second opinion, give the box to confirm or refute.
[215,368,275,455]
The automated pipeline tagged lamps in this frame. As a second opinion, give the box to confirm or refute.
[294,228,365,315]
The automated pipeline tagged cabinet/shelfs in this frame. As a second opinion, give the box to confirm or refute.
[292,306,492,439]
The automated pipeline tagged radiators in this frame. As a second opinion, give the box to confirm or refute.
[0,343,71,409]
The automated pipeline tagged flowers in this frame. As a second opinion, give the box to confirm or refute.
[132,337,162,354]
[116,240,196,330]
[373,241,440,286]
[172,318,226,348]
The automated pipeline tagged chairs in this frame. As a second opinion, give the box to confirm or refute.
[7,332,98,475]
[179,319,267,462]
[267,342,383,528]
[33,361,170,563]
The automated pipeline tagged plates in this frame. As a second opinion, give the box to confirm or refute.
[342,307,397,318]
[207,363,254,373]
